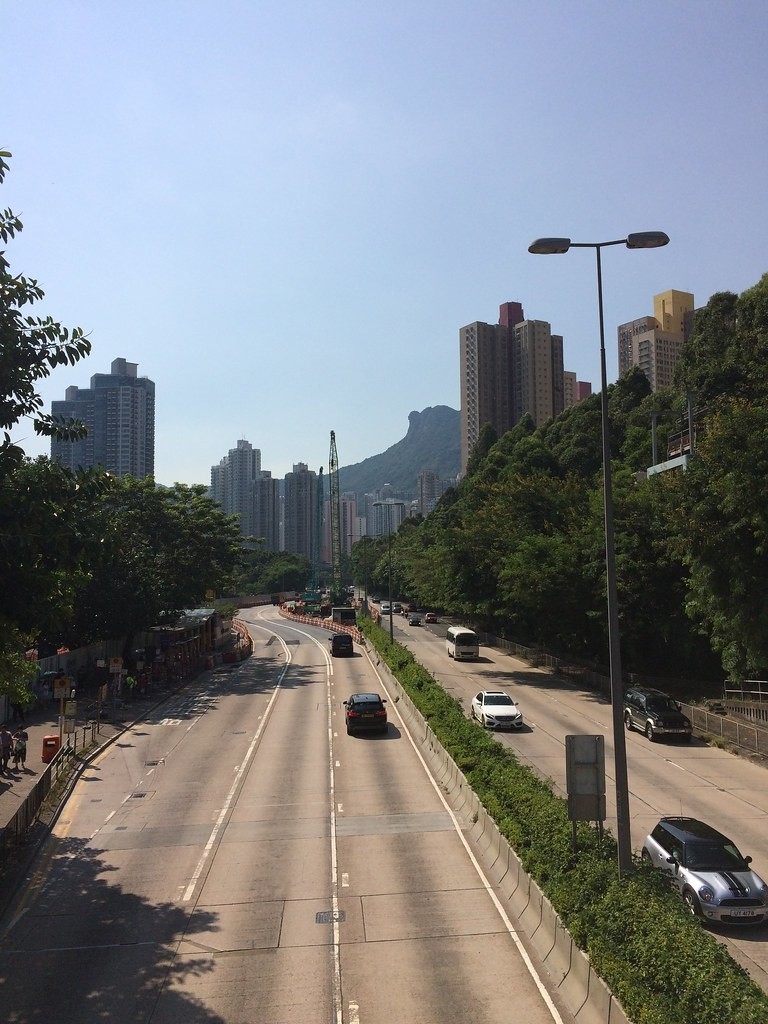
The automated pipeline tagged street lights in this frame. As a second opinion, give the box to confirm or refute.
[528,230,669,879]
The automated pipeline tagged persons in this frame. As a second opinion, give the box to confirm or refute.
[43,665,85,709]
[10,702,25,723]
[0,724,28,773]
[139,674,148,700]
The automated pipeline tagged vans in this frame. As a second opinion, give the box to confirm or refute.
[328,633,353,657]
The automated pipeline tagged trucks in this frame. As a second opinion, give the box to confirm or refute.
[270,592,288,606]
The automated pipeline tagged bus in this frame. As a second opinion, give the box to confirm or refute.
[445,626,479,661]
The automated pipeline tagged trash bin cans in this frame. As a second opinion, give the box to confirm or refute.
[41,735,61,763]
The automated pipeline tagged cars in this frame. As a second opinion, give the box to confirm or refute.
[424,612,437,623]
[371,596,406,616]
[642,816,768,926]
[470,691,523,731]
[408,615,422,626]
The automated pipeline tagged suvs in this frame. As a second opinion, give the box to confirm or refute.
[343,693,388,735]
[622,687,693,743]
